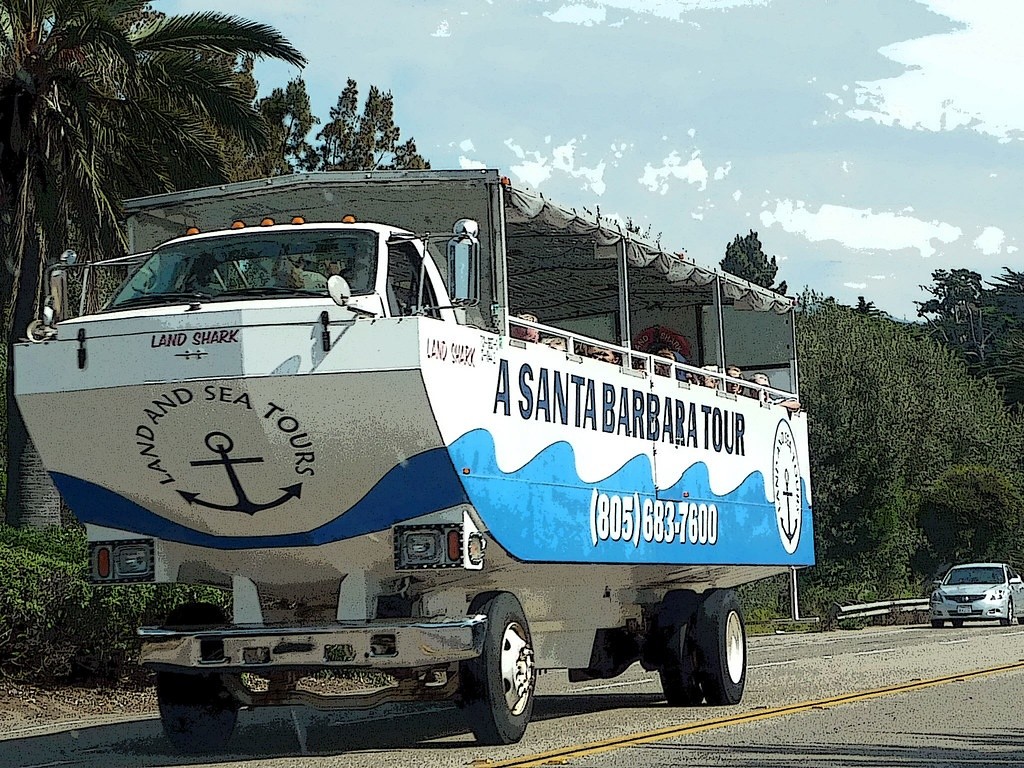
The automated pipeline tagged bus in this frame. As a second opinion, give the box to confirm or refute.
[12,165,817,751]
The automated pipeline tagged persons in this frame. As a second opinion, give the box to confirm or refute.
[280,259,321,290]
[508,313,623,366]
[634,349,799,409]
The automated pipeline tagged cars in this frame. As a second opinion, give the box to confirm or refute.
[928,563,1024,627]
